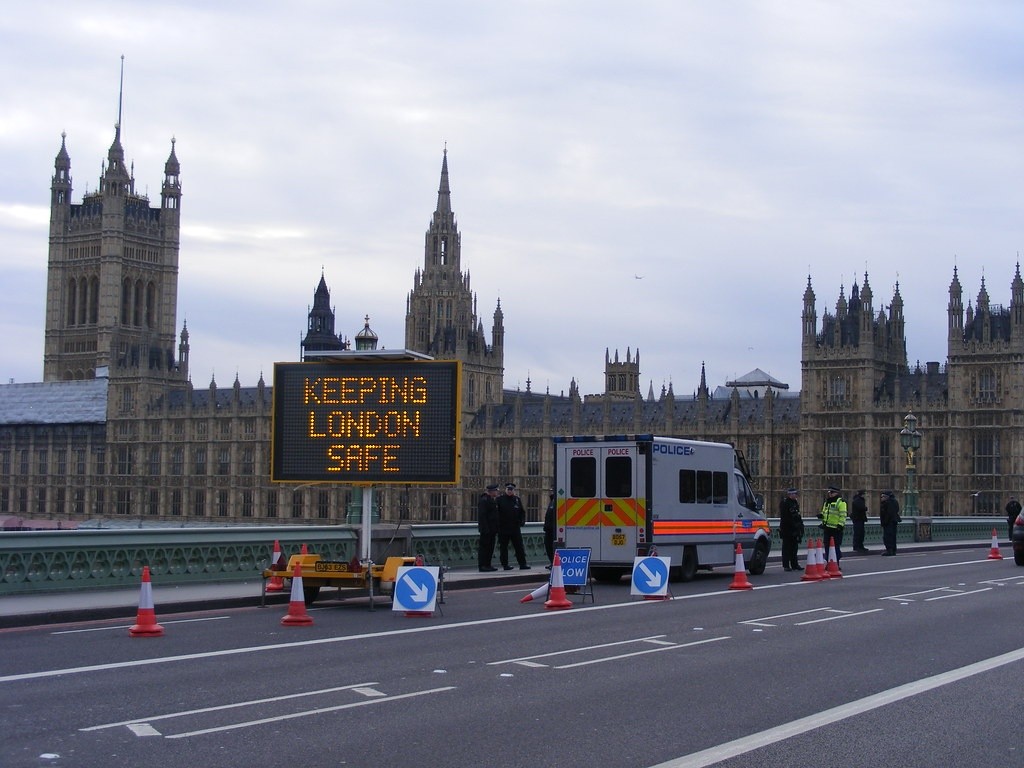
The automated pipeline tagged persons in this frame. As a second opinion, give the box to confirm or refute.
[881,493,885,519]
[495,483,531,570]
[779,488,805,571]
[544,485,556,570]
[882,492,899,557]
[852,490,869,552]
[817,487,847,569]
[1006,496,1022,540]
[478,484,498,571]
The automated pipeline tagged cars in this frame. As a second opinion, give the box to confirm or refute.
[1011,507,1024,566]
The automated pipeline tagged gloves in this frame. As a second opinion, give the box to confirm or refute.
[837,524,842,533]
[865,507,868,511]
[816,514,823,519]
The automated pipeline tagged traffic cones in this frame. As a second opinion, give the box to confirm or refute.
[520,581,581,603]
[728,542,754,590]
[281,561,314,627]
[545,553,573,611]
[263,539,283,592]
[642,554,671,600]
[302,543,307,555]
[128,566,165,637]
[403,557,432,617]
[987,527,1004,560]
[799,536,842,580]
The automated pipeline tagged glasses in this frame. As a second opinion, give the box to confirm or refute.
[506,488,513,490]
[880,496,883,498]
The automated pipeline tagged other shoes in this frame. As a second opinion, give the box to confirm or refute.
[545,564,552,570]
[505,565,513,570]
[479,567,497,571]
[519,564,531,569]
[853,547,869,552]
[881,551,896,556]
[784,566,804,572]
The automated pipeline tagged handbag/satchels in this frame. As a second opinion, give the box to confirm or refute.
[897,514,902,522]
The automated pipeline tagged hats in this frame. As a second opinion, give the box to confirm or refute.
[505,483,516,489]
[829,486,841,492]
[486,484,500,491]
[858,489,866,494]
[788,488,797,494]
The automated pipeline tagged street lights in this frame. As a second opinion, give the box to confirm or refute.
[345,315,381,524]
[899,410,922,516]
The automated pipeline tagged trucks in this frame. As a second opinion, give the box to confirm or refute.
[552,432,773,584]
